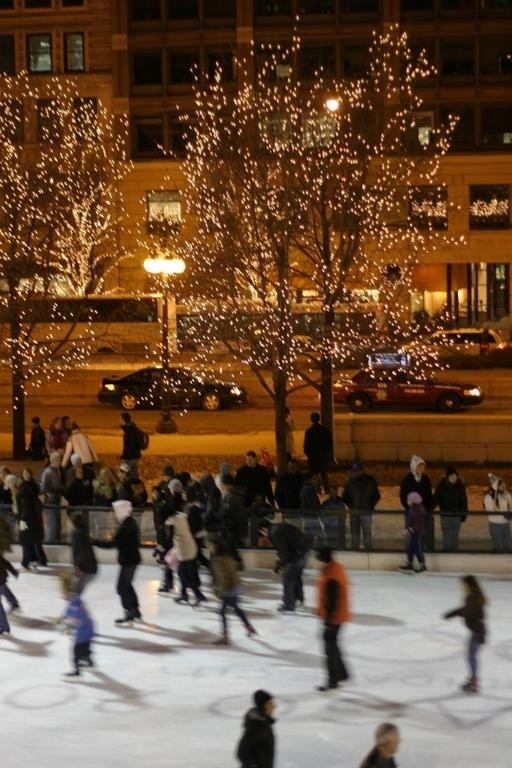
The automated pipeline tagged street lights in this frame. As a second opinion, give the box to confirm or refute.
[142,246,198,435]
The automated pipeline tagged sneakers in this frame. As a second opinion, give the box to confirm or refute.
[214,634,227,644]
[245,624,254,633]
[65,662,94,677]
[114,610,141,622]
[396,564,427,574]
[276,606,294,613]
[158,586,208,607]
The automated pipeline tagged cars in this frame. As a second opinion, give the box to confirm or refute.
[96,364,248,416]
[314,363,485,415]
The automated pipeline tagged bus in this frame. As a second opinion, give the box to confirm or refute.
[0,291,179,363]
[169,289,390,366]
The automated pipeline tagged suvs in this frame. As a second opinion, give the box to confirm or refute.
[398,324,509,367]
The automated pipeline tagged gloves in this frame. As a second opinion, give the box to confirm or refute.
[398,527,414,539]
[19,520,28,531]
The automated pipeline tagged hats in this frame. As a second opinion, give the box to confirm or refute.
[120,464,131,471]
[489,474,502,506]
[315,547,333,562]
[168,479,183,491]
[220,464,231,475]
[410,454,426,482]
[254,690,272,705]
[407,492,423,504]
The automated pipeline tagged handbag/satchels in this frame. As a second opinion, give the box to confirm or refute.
[138,431,149,450]
